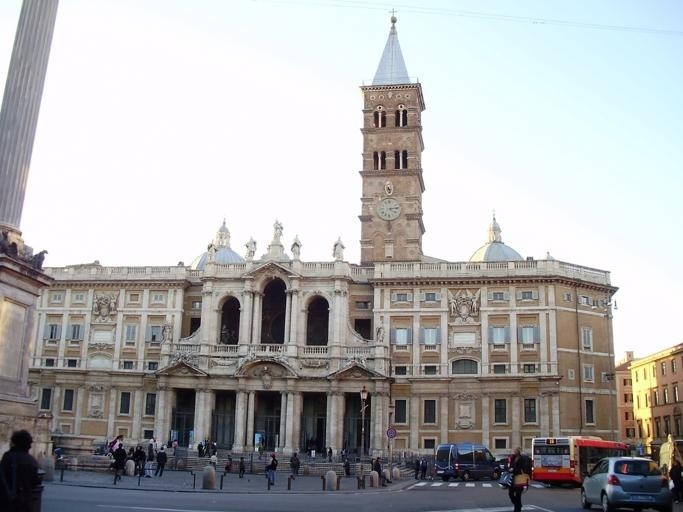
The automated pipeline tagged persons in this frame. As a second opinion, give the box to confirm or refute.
[491,449,532,512]
[244,236,257,260]
[291,235,303,258]
[321,447,327,459]
[327,447,333,462]
[290,452,300,479]
[92,295,100,313]
[109,435,179,481]
[342,447,352,477]
[224,457,234,477]
[448,295,458,316]
[331,236,346,261]
[471,295,478,315]
[269,452,278,485]
[669,460,683,504]
[374,457,392,487]
[239,457,246,479]
[0,430,43,512]
[420,459,428,480]
[194,440,218,470]
[208,239,219,261]
[109,294,117,312]
[414,457,420,480]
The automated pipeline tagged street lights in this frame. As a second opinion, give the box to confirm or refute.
[358,384,370,471]
[592,296,619,441]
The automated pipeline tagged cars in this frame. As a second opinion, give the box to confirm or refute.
[581,455,672,511]
[491,452,532,480]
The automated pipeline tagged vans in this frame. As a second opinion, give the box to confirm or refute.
[433,443,501,479]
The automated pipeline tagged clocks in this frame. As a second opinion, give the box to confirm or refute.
[376,198,402,222]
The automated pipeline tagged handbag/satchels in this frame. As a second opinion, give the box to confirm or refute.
[514,474,530,486]
[500,472,513,487]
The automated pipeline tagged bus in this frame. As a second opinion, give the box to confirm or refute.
[531,435,630,487]
